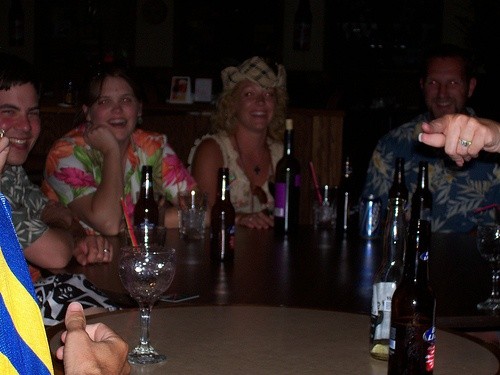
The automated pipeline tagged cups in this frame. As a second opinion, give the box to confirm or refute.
[309,186,338,231]
[176,190,207,240]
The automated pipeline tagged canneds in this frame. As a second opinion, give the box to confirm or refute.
[360,194,383,240]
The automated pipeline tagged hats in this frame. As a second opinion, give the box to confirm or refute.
[221,56,286,89]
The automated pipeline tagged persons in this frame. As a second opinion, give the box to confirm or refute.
[360,48,500,236]
[0,64,212,375]
[187,56,300,233]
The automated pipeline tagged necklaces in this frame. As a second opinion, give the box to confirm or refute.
[234,132,266,175]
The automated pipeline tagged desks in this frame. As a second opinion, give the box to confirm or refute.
[43,224,500,375]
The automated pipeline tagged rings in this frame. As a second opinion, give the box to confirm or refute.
[460,138,472,149]
[103,247,111,253]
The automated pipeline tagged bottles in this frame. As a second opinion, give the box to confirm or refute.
[408,161,432,246]
[273,118,303,237]
[336,155,358,232]
[388,219,436,375]
[368,196,404,361]
[7,0,25,48]
[387,160,409,211]
[133,165,160,245]
[208,167,237,261]
[291,0,313,51]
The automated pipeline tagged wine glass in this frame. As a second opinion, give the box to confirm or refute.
[476,223,500,310]
[116,245,176,365]
